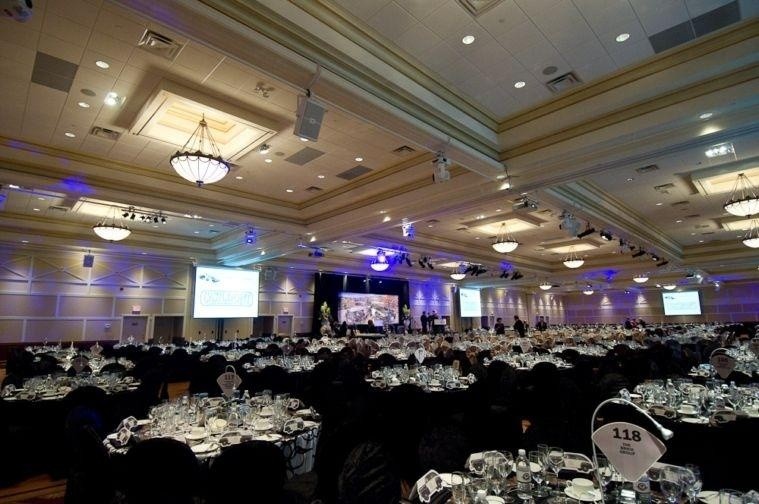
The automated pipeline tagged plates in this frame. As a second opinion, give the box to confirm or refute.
[191,443,218,453]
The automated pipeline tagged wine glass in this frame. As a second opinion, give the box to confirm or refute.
[619,367,759,423]
[1,371,141,402]
[362,360,475,390]
[449,440,759,503]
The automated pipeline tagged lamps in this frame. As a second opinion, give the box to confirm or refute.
[168,116,231,191]
[740,220,758,250]
[489,225,521,256]
[448,265,468,284]
[88,206,134,244]
[722,171,758,219]
[537,275,678,296]
[561,248,587,271]
[368,256,392,273]
[123,207,173,227]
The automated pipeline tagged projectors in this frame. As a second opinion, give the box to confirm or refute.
[512,199,537,214]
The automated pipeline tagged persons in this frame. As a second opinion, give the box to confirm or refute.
[494,317,506,336]
[513,314,530,335]
[366,320,394,336]
[421,310,441,336]
[625,317,646,330]
[535,315,550,333]
[339,321,355,336]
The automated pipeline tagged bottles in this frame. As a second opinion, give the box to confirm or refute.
[516,448,533,500]
[635,471,651,503]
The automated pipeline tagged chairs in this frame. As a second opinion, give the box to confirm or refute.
[3,318,757,502]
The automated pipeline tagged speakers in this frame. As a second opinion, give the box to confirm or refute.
[294,96,326,143]
[82,254,94,267]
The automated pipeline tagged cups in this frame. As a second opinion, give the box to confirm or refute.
[149,390,287,445]
